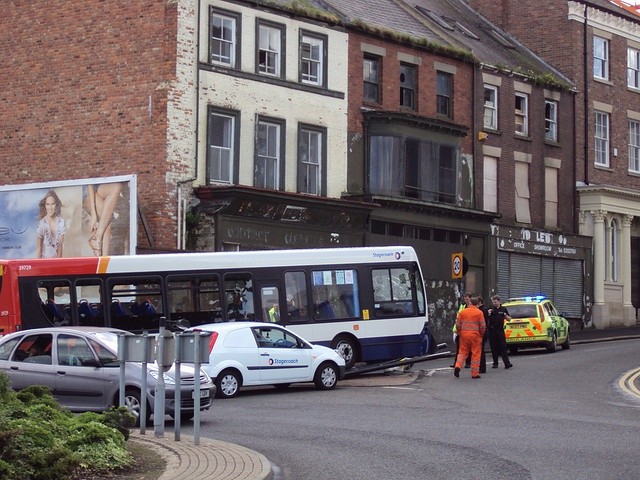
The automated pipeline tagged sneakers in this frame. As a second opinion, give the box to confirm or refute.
[450,362,455,366]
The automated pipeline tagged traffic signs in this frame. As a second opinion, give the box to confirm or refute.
[452,252,463,279]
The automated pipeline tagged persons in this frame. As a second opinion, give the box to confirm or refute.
[454,296,487,379]
[81,182,130,257]
[487,294,513,368]
[36,190,66,259]
[474,295,487,373]
[451,292,473,367]
[269,303,281,322]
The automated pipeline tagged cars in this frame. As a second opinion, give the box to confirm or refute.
[0,326,216,427]
[174,321,346,397]
[501,295,571,354]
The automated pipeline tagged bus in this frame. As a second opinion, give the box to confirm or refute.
[0,246,434,371]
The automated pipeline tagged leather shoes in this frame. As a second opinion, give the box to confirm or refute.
[472,376,481,378]
[505,364,512,369]
[454,368,459,377]
[493,365,497,368]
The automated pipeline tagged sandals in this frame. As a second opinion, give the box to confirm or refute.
[88,236,103,256]
